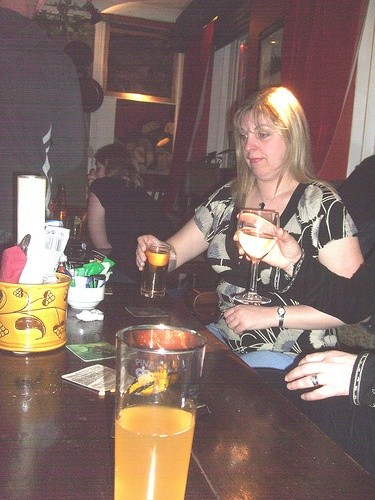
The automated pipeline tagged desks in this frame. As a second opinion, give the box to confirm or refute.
[1,248,375,500]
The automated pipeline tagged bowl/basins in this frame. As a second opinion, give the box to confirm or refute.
[67,277,105,310]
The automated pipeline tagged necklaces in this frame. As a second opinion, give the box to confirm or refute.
[256,182,277,210]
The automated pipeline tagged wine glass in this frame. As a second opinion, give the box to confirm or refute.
[235,209,281,304]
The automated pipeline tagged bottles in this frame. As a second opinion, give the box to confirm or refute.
[51,184,67,226]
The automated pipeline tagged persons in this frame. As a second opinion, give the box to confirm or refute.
[233,209,375,474]
[0,0,88,250]
[88,144,169,299]
[137,87,365,370]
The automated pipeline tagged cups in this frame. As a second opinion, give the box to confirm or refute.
[140,242,170,299]
[111,324,207,499]
[1,272,73,354]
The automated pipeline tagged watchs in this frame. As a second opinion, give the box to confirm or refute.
[277,305,286,327]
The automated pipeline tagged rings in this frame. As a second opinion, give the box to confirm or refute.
[310,375,321,388]
